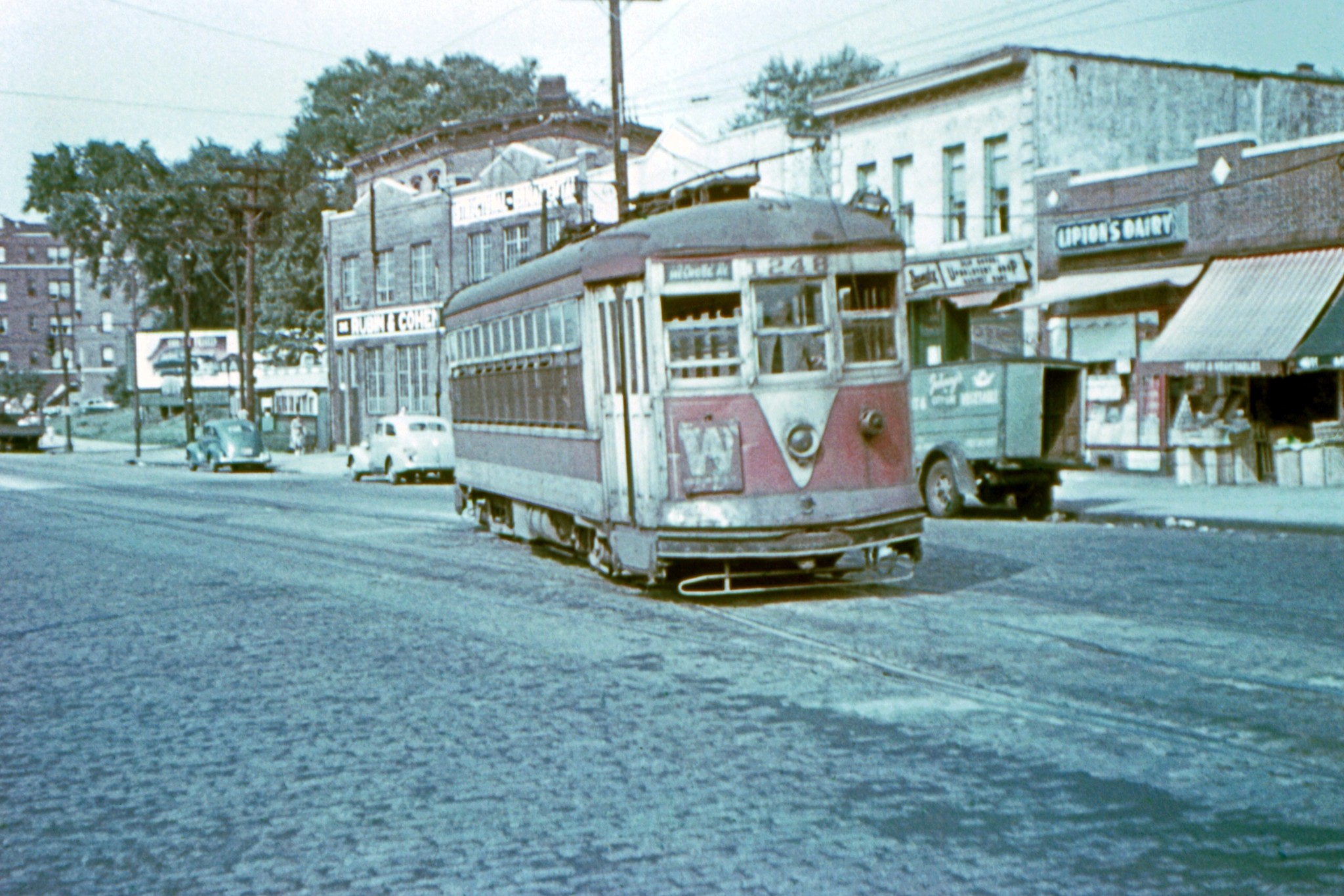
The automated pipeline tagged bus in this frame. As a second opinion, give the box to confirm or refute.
[438,189,929,596]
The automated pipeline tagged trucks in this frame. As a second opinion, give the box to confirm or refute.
[910,358,1089,520]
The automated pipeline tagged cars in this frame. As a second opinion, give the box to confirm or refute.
[186,417,271,473]
[346,413,454,485]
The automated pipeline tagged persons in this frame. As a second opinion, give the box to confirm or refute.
[260,406,276,453]
[237,405,251,433]
[289,415,307,455]
